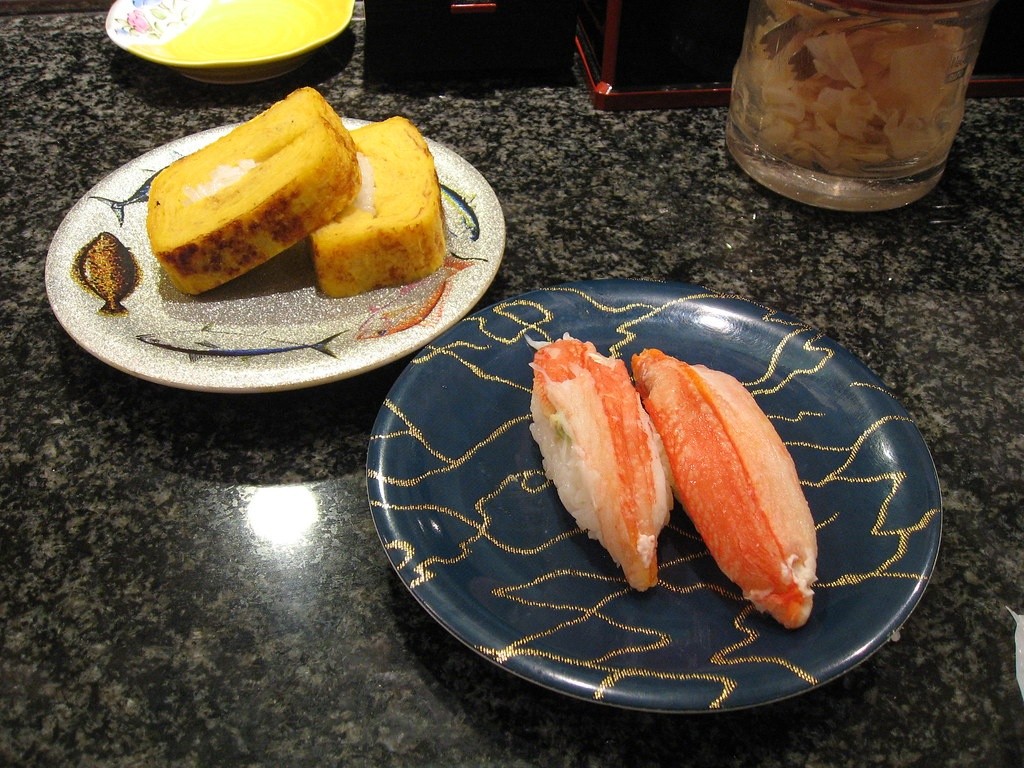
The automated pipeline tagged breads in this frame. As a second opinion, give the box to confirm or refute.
[305,115,450,297]
[144,85,363,296]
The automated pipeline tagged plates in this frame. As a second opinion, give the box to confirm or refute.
[106,0,354,84]
[44,119,506,393]
[365,278,943,714]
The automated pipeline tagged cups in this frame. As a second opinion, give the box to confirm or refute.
[726,0,994,211]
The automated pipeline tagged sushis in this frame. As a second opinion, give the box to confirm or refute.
[518,332,819,630]
